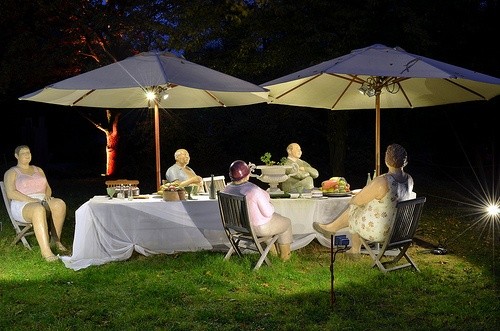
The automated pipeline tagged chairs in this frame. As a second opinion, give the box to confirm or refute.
[105,179,139,188]
[202,175,227,193]
[0,182,35,251]
[217,189,279,271]
[360,197,427,274]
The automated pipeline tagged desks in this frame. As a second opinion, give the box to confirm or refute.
[56,189,416,272]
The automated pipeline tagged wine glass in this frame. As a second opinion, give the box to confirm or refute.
[107,188,115,200]
[295,187,305,198]
[184,186,193,200]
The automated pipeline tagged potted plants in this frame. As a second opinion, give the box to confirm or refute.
[250,152,299,194]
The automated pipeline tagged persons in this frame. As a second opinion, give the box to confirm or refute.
[222,161,292,261]
[4,146,66,262]
[166,149,203,187]
[313,144,414,259]
[282,143,318,191]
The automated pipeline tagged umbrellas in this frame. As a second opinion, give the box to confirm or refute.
[262,44,500,174]
[18,51,273,192]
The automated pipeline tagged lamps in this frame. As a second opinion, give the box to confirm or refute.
[334,234,350,249]
[162,91,170,100]
[358,81,376,97]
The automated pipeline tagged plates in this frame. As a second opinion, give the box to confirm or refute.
[326,192,352,196]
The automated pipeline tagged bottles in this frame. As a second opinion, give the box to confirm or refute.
[120,184,125,199]
[367,173,372,184]
[208,175,216,199]
[373,170,376,179]
[127,184,133,201]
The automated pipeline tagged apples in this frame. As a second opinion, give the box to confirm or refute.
[321,180,346,194]
